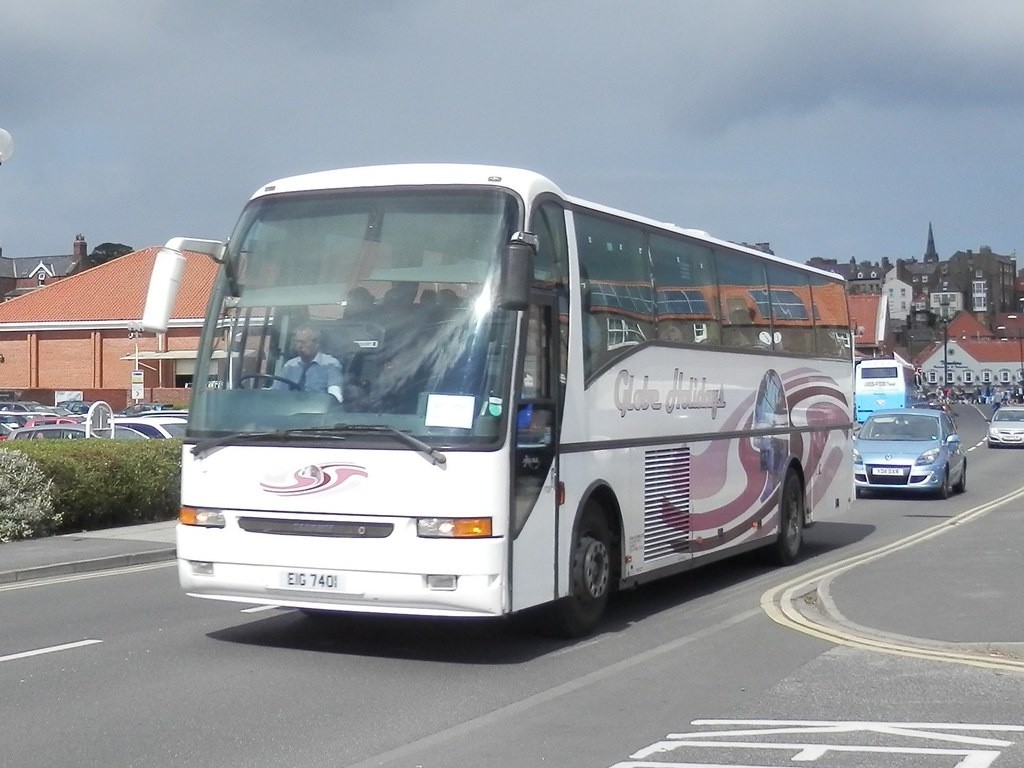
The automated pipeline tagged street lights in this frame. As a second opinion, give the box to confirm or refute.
[996,315,1024,371]
[128,321,145,404]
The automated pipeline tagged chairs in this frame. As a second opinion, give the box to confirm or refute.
[318,327,363,411]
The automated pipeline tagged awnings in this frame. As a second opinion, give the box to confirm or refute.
[120,350,255,364]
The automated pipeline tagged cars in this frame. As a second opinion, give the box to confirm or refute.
[0,400,189,441]
[83,417,188,439]
[853,408,967,499]
[985,407,1024,448]
[7,424,149,440]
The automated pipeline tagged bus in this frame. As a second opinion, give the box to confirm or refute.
[142,162,856,634]
[856,358,922,425]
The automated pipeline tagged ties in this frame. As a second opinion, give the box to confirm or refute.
[298,362,315,390]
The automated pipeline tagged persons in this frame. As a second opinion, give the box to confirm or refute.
[937,383,1024,410]
[335,279,470,410]
[260,325,345,405]
